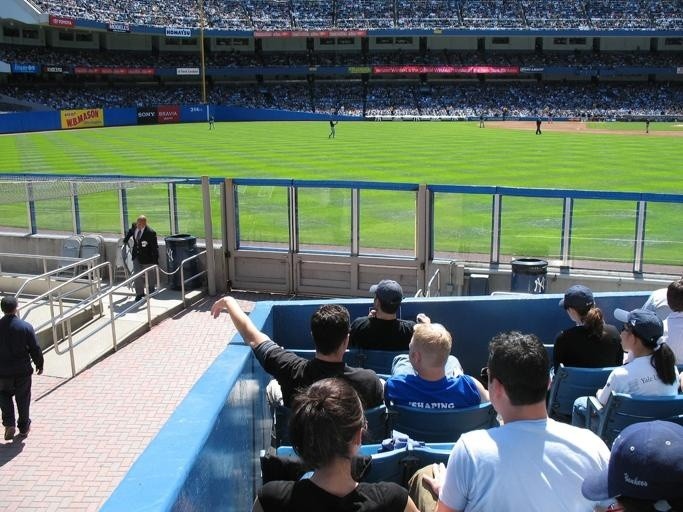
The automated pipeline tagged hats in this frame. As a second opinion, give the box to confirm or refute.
[368,279,404,306]
[613,306,665,341]
[582,420,683,503]
[558,285,595,310]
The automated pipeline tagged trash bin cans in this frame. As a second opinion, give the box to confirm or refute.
[510,257,548,293]
[164,234,202,290]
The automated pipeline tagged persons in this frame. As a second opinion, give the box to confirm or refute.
[329,120,336,138]
[209,114,215,130]
[123,215,159,302]
[0,296,44,441]
[479,114,484,128]
[536,117,542,135]
[646,118,649,133]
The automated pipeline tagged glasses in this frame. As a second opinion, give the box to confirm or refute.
[479,367,490,382]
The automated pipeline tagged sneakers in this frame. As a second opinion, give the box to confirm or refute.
[19,418,31,436]
[4,426,16,440]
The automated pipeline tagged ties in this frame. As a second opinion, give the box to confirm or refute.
[136,230,141,241]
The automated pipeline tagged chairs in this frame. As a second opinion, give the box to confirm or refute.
[259,348,500,485]
[542,344,682,450]
[58,233,133,284]
